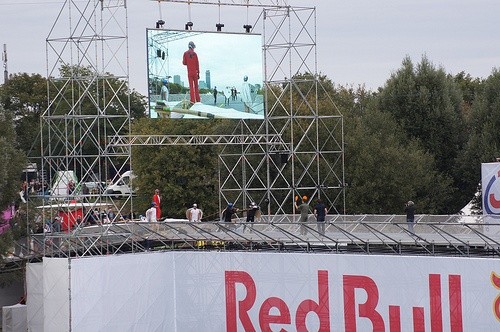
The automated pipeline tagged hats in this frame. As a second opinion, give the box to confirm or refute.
[188,41,196,48]
[151,202,157,206]
[244,75,249,81]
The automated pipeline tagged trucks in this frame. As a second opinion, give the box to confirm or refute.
[106,170,139,201]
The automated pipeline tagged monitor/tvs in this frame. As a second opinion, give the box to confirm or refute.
[145,28,265,120]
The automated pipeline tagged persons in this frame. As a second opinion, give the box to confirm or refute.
[295,199,313,236]
[161,80,169,101]
[246,202,260,222]
[239,75,252,112]
[214,86,217,103]
[404,201,415,236]
[182,41,200,103]
[224,86,237,106]
[127,210,136,219]
[14,188,27,211]
[314,198,327,237]
[225,202,239,222]
[10,209,62,257]
[77,208,117,225]
[146,188,161,252]
[22,179,43,194]
[189,204,200,222]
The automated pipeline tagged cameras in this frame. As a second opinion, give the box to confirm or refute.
[404,204,408,206]
